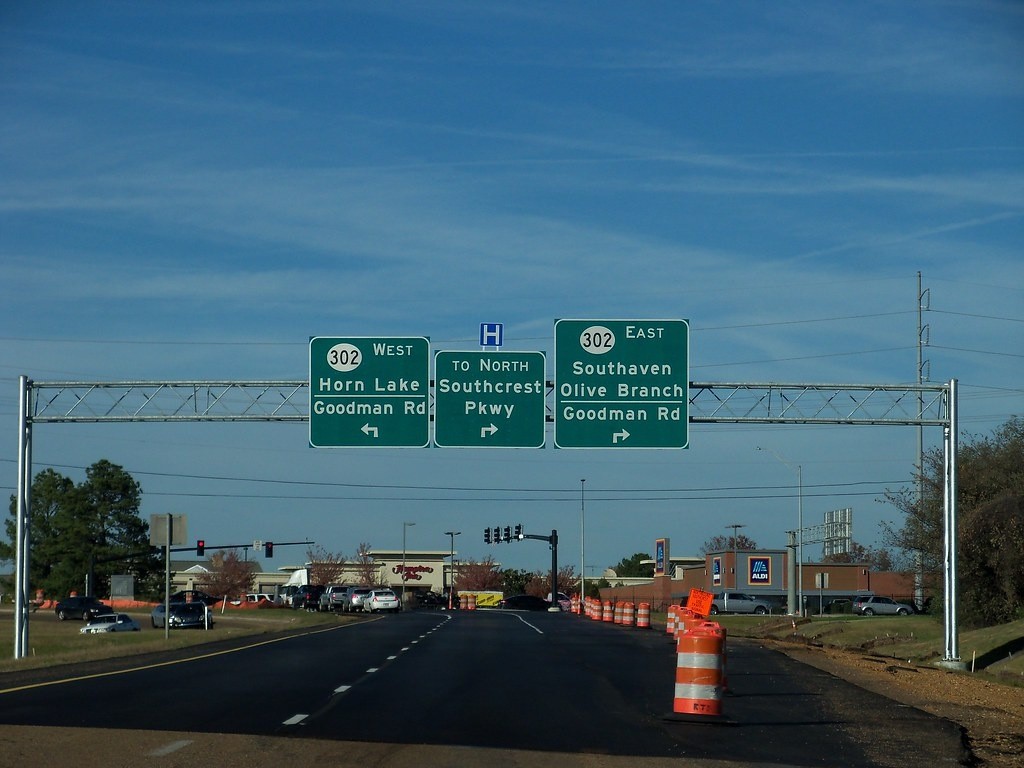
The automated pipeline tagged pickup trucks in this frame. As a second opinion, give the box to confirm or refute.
[318,584,354,610]
[710,590,775,615]
[541,591,572,612]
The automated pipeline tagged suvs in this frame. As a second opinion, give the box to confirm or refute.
[241,584,325,611]
[852,595,915,617]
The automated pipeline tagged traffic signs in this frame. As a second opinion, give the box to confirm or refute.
[309,334,431,449]
[433,350,546,450]
[553,318,690,450]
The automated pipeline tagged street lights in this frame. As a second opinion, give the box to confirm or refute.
[724,522,747,589]
[444,531,462,593]
[581,478,586,602]
[402,521,416,612]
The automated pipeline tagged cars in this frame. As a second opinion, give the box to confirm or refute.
[833,599,851,607]
[342,587,373,613]
[54,596,115,622]
[361,588,399,615]
[413,591,461,611]
[150,596,214,630]
[79,612,142,636]
[497,595,550,612]
[165,589,222,606]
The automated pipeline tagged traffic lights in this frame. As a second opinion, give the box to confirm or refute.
[504,526,510,543]
[484,527,490,544]
[266,542,272,557]
[515,524,521,541]
[494,526,500,544]
[197,540,204,556]
